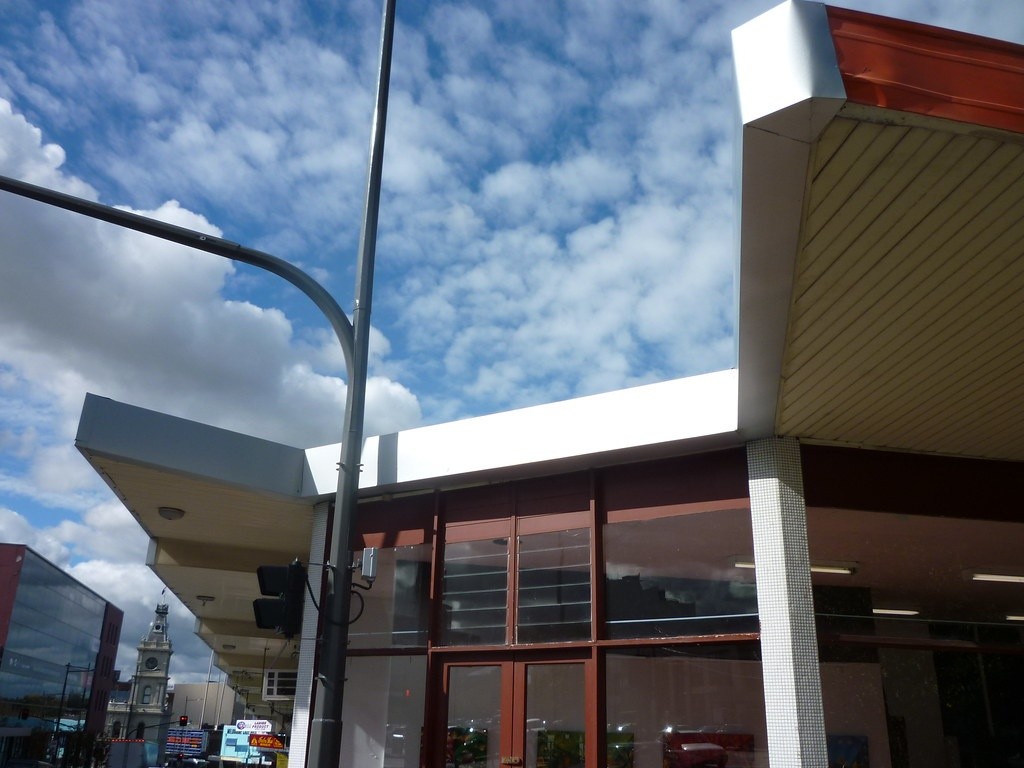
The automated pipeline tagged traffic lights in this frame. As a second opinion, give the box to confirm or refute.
[180,716,188,727]
[254,564,303,637]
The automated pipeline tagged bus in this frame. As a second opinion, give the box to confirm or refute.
[167,758,211,768]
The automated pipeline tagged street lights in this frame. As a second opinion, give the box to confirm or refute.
[54,663,96,734]
